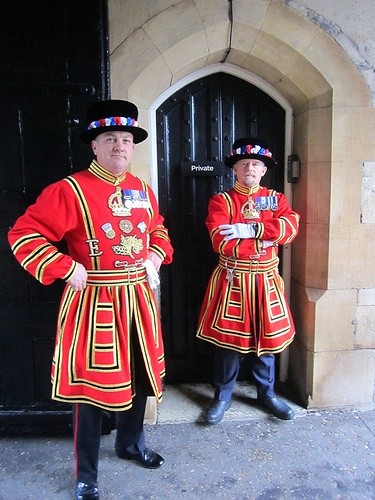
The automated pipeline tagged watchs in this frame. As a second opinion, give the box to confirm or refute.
[253,223,258,234]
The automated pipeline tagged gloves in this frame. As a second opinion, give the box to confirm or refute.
[219,223,255,241]
[142,259,160,290]
[263,240,279,250]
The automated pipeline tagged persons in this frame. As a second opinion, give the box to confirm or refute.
[196,137,300,424]
[8,99,173,500]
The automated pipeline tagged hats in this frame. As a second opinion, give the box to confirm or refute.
[225,137,275,169]
[80,100,148,145]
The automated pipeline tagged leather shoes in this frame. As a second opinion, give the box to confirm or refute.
[257,396,295,420]
[204,399,232,424]
[75,482,99,500]
[115,447,164,469]
